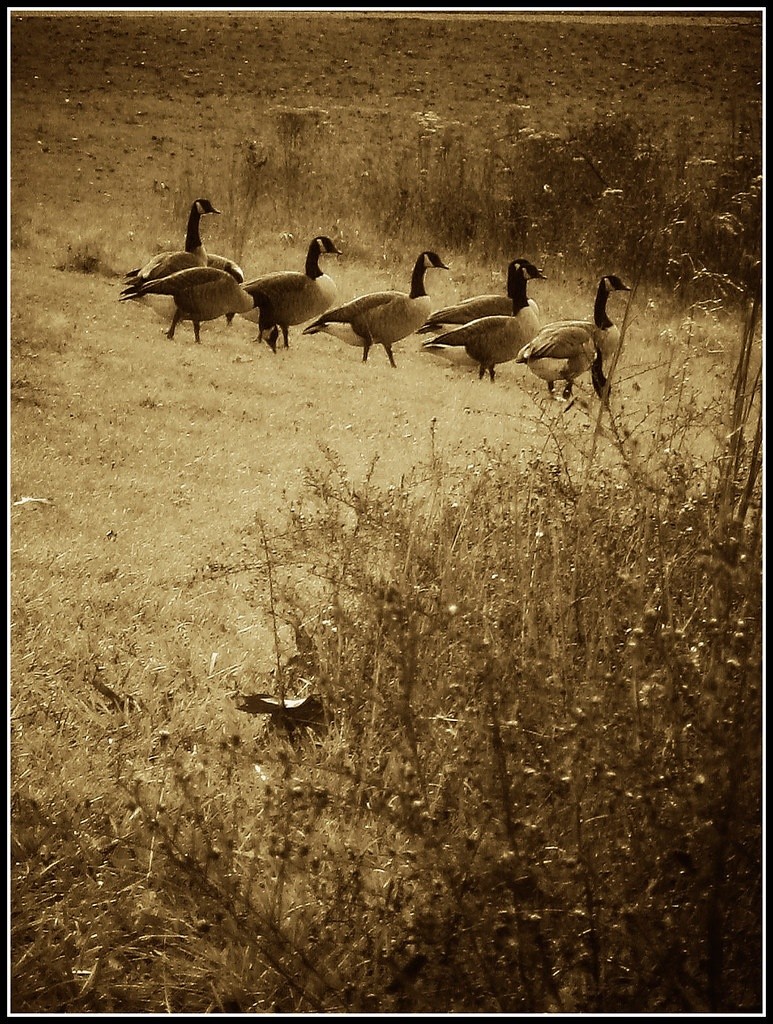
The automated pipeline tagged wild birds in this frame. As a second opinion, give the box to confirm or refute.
[119,197,633,400]
